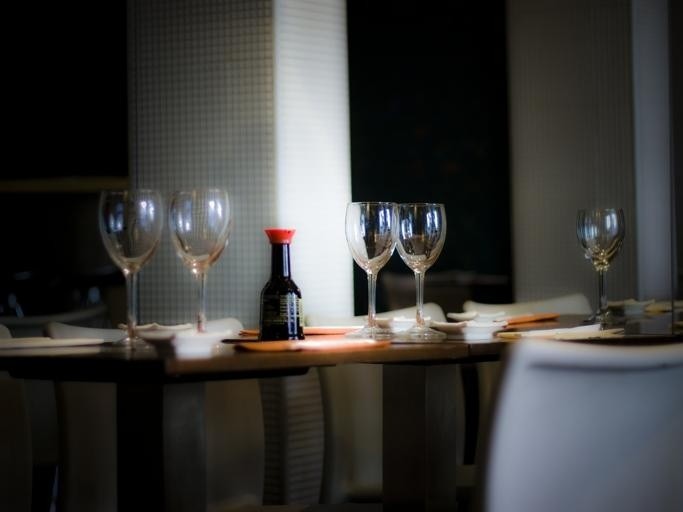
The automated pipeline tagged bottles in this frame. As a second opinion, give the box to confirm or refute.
[260,228,303,340]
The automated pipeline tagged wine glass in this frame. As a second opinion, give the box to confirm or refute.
[576,207,625,324]
[346,201,448,341]
[99,187,231,348]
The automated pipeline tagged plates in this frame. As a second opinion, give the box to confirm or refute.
[427,311,508,340]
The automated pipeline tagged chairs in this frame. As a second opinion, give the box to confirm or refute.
[480,334,682,511]
